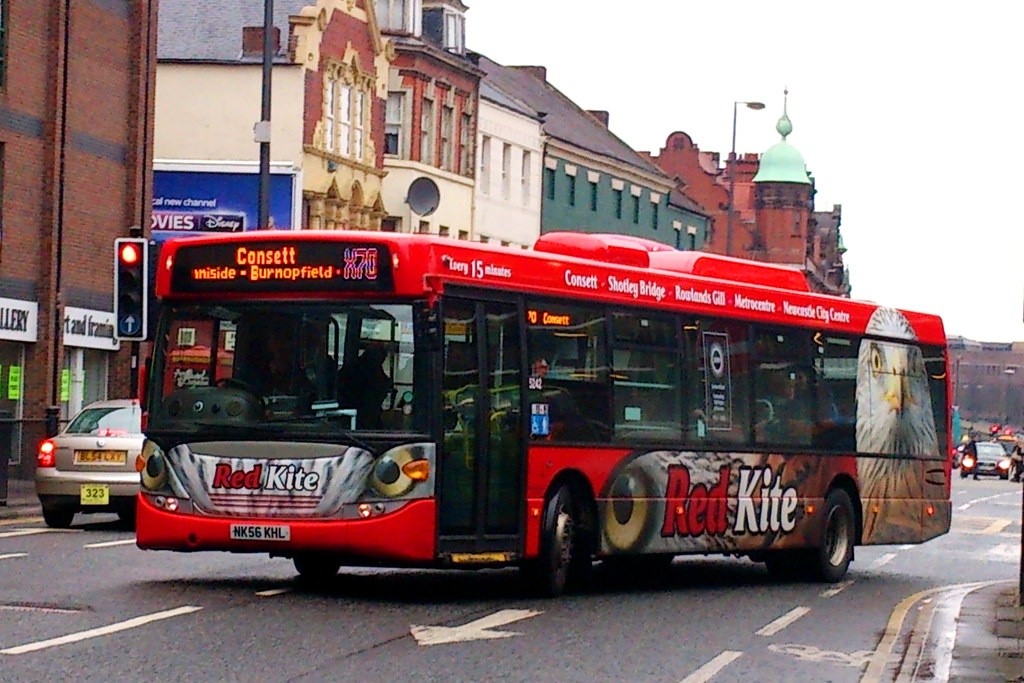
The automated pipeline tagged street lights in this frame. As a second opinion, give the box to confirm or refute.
[725,99,766,257]
[954,354,962,406]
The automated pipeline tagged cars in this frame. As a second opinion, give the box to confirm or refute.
[31,398,147,529]
[952,431,1024,480]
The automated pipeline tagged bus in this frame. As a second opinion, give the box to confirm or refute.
[135,226,956,597]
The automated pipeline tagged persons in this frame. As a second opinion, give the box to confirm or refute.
[962,440,980,479]
[333,343,392,413]
[1010,442,1024,482]
[786,365,819,421]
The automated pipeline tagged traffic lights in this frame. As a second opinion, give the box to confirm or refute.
[111,236,150,343]
